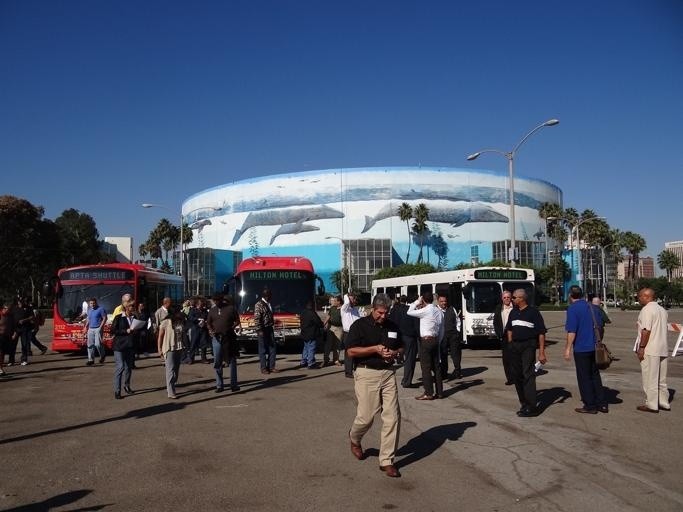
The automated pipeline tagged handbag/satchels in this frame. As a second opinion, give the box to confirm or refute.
[595,342,613,369]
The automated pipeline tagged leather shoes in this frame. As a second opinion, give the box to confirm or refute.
[415,393,433,400]
[351,440,363,460]
[517,404,670,417]
[380,465,401,477]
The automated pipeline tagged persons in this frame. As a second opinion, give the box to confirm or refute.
[389,292,462,400]
[590,297,611,343]
[506,289,547,417]
[300,300,324,369]
[319,295,344,368]
[79,292,241,399]
[565,286,608,414]
[340,292,361,378]
[493,291,513,385]
[254,290,280,374]
[0,297,48,376]
[345,293,406,478]
[632,287,672,413]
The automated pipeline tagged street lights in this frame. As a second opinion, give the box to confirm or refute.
[583,243,619,314]
[546,216,607,288]
[140,203,221,275]
[466,118,559,268]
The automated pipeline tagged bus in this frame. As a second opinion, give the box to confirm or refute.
[40,263,185,353]
[370,266,535,351]
[222,256,324,352]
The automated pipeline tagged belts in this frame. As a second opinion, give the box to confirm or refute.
[425,337,432,340]
